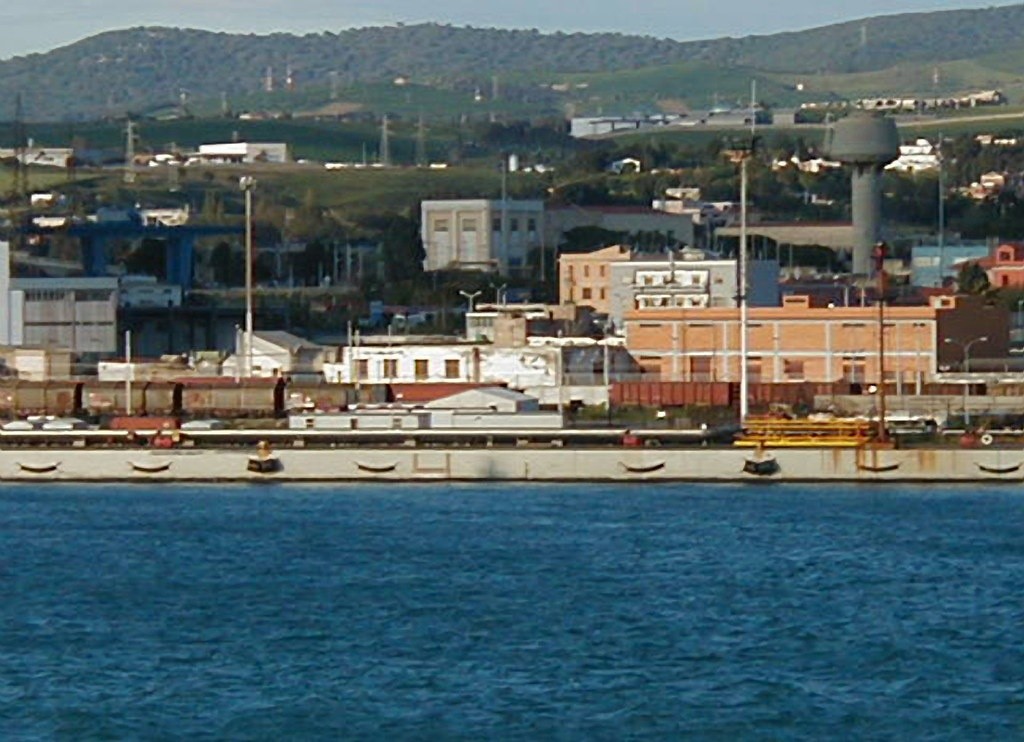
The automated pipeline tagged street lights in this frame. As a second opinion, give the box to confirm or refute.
[945,338,990,432]
[719,132,764,432]
[239,176,257,380]
[458,289,483,315]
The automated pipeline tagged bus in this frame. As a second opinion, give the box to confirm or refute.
[862,414,939,438]
